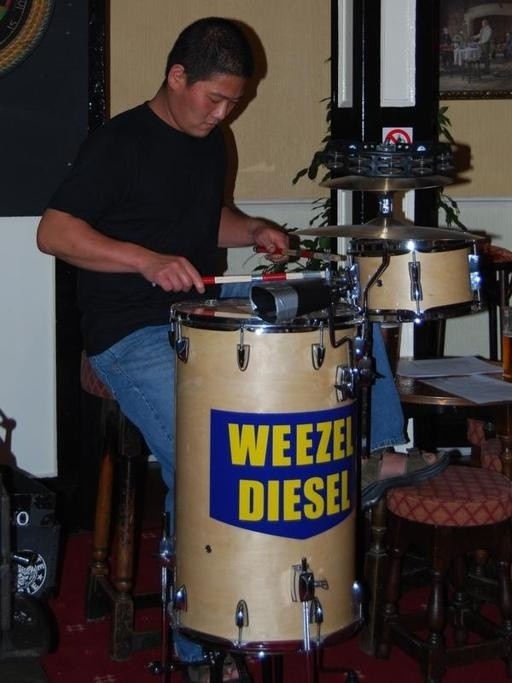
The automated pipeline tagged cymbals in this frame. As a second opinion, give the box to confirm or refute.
[318,176,471,192]
[287,225,484,240]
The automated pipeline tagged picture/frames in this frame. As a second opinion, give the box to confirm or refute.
[431,1,511,102]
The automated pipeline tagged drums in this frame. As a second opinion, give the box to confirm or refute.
[169,299,365,657]
[348,238,481,322]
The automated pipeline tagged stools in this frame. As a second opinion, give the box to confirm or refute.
[372,418,512,683]
[71,356,172,662]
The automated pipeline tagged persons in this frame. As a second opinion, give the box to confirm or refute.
[469,18,491,74]
[33,12,460,682]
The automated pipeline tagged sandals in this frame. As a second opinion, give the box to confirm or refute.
[360,446,451,506]
